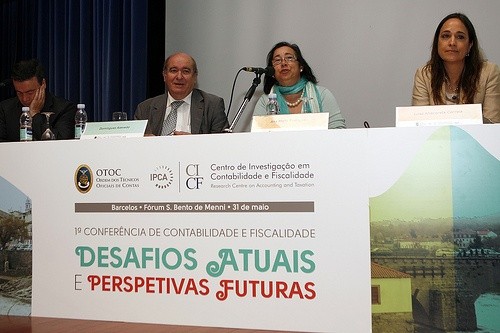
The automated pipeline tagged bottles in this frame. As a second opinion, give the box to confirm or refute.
[19,107,32,141]
[74,104,87,139]
[266,93,280,115]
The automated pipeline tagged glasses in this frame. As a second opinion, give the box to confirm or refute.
[272,56,297,64]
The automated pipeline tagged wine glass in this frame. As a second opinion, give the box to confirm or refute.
[41,112,57,140]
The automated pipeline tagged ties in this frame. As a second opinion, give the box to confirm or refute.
[160,101,185,136]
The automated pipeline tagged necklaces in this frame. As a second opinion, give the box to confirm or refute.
[282,89,304,106]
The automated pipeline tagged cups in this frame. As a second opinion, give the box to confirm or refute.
[112,112,128,121]
[296,98,318,113]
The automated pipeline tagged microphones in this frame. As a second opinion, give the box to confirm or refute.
[243,66,275,77]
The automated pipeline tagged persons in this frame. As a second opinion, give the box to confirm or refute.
[251,42,346,130]
[0,59,77,142]
[134,54,229,136]
[412,13,500,124]
